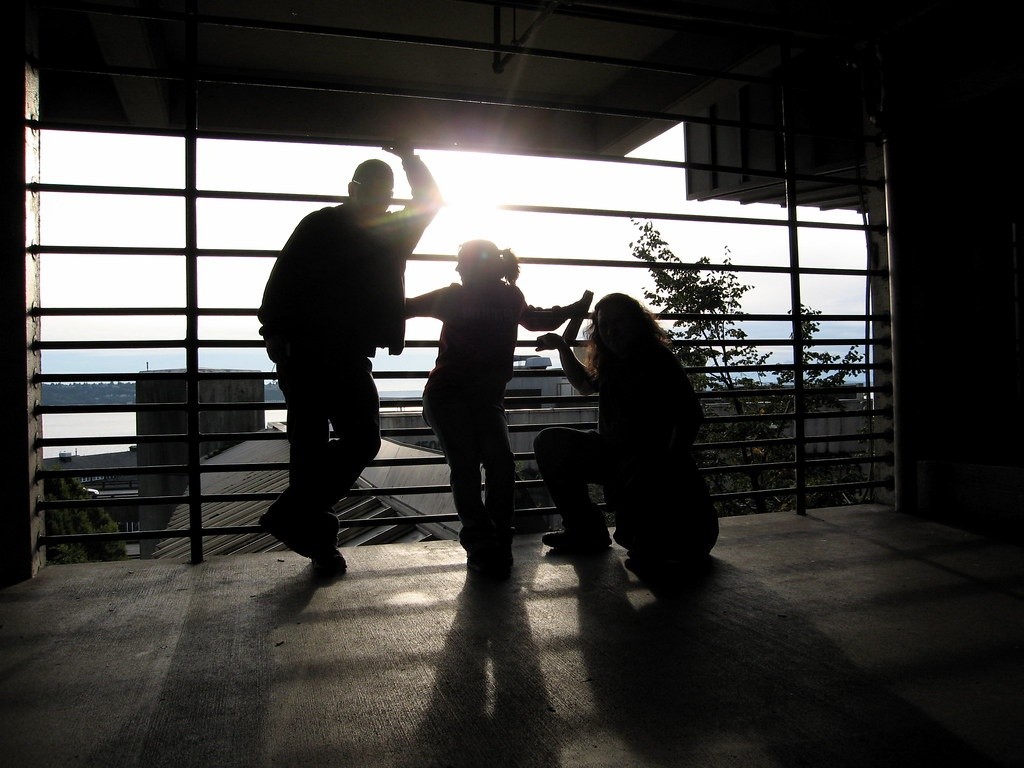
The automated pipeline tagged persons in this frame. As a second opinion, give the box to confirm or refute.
[532,292,720,573]
[399,239,580,577]
[257,135,443,574]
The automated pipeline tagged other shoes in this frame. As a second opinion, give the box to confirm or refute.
[312,550,346,577]
[258,514,339,558]
[542,528,612,547]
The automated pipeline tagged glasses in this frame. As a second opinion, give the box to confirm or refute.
[353,180,393,198]
